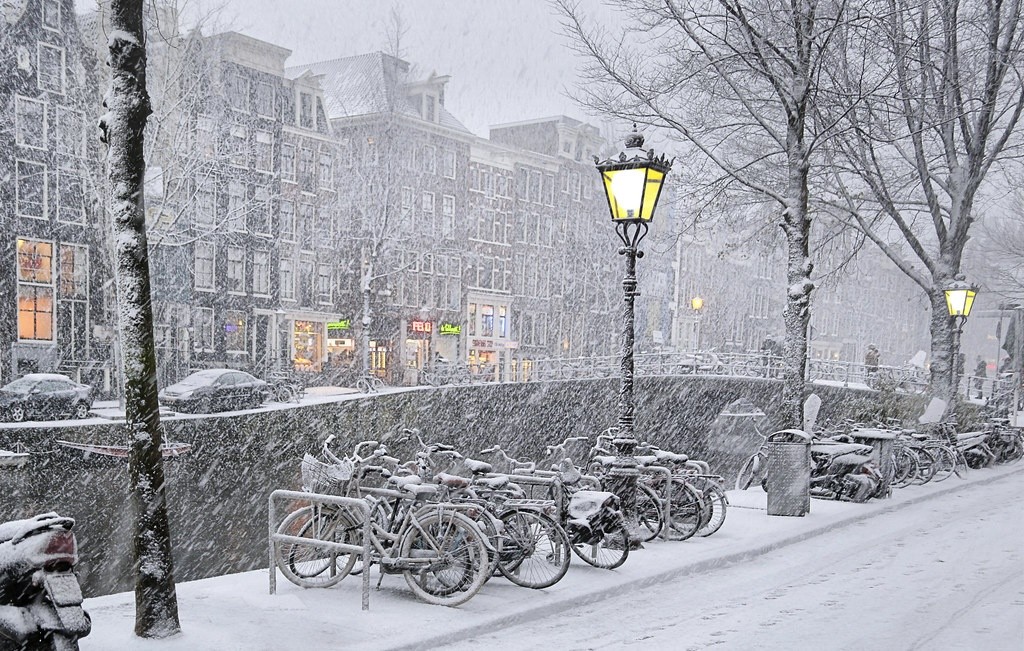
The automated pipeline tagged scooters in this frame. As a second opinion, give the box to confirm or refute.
[1,509,91,651]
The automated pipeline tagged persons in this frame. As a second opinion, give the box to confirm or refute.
[974,355,987,399]
[864,345,881,373]
[957,353,965,382]
[763,337,782,378]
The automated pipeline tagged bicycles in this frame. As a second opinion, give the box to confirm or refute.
[355,367,385,395]
[736,413,1024,504]
[274,427,732,607]
[417,337,932,393]
[277,383,306,402]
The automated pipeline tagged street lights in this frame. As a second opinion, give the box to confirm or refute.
[943,273,982,432]
[594,122,675,550]
[690,297,703,374]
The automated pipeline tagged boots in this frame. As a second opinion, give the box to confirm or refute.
[975,393,982,399]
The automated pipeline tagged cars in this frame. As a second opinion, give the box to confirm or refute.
[156,368,271,414]
[0,374,95,421]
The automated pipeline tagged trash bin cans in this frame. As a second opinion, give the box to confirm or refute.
[851,430,895,497]
[765,429,812,517]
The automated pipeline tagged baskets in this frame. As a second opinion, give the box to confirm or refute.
[301,456,350,496]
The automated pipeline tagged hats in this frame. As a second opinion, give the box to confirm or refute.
[977,355,981,361]
[766,335,771,339]
[869,345,875,349]
